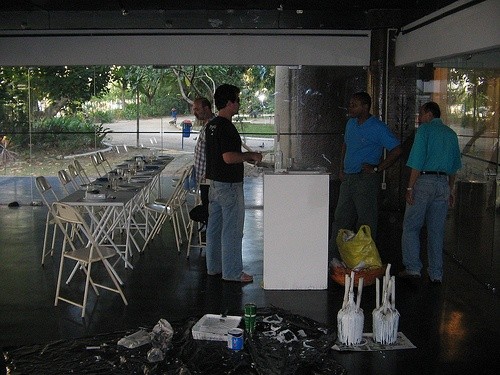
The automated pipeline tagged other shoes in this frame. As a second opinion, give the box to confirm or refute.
[207,271,222,275]
[223,271,253,283]
[399,271,420,279]
[428,276,442,284]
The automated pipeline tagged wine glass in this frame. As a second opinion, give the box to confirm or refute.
[85,149,161,193]
[253,150,262,168]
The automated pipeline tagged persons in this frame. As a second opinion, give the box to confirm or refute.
[401,102,461,286]
[331,92,405,259]
[171,84,263,281]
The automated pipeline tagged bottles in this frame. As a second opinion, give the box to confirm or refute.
[274,141,283,171]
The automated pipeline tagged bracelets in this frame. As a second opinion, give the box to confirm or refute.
[407,188,413,191]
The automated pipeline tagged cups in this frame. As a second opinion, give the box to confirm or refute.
[244,303,258,332]
[285,157,294,169]
[183,123,192,137]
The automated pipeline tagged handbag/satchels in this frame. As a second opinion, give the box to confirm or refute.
[336,225,384,270]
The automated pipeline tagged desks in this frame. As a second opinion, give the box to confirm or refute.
[263,166,329,290]
[55,156,174,284]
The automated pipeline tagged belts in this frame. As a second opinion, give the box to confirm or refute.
[419,171,446,176]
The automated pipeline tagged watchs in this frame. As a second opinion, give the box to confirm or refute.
[373,166,379,173]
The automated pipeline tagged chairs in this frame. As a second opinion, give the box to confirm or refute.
[35,151,208,317]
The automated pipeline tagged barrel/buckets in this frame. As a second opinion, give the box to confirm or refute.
[227,328,244,350]
